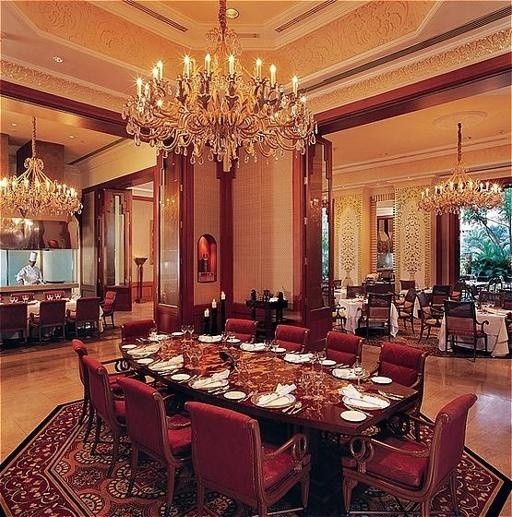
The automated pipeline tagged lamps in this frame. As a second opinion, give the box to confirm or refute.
[122,1,318,172]
[0,116,83,223]
[418,123,502,216]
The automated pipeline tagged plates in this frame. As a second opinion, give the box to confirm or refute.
[121,332,393,423]
[46,281,65,284]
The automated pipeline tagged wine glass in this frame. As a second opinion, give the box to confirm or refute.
[148,324,363,399]
[10,293,61,303]
[355,293,364,300]
[481,301,495,316]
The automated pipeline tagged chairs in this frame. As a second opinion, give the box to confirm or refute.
[73,302,429,515]
[0,274,117,346]
[340,393,478,517]
[324,275,511,362]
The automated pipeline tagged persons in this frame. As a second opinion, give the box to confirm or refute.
[16,252,44,286]
